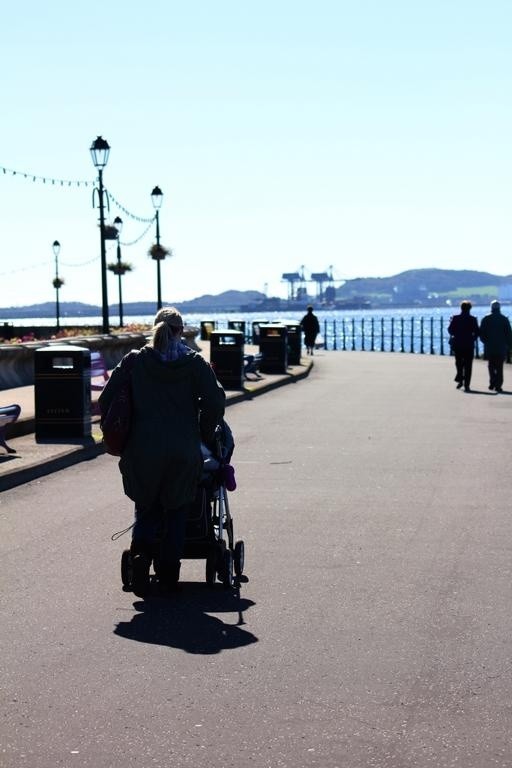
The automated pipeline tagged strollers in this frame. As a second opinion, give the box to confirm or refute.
[119,422,245,588]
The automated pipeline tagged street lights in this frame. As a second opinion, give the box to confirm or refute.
[110,213,128,326]
[147,184,166,312]
[52,238,65,326]
[89,133,113,333]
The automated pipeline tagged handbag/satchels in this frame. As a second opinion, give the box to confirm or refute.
[101,394,132,456]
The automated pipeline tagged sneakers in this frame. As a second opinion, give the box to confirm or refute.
[130,554,151,599]
[456,383,469,392]
[489,382,503,392]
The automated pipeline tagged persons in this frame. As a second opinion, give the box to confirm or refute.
[479,300,512,392]
[448,299,479,391]
[301,305,320,356]
[98,307,226,595]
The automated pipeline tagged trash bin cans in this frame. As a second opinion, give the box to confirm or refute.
[277,322,301,366]
[260,324,287,374]
[210,330,246,390]
[202,321,270,344]
[35,346,91,443]
